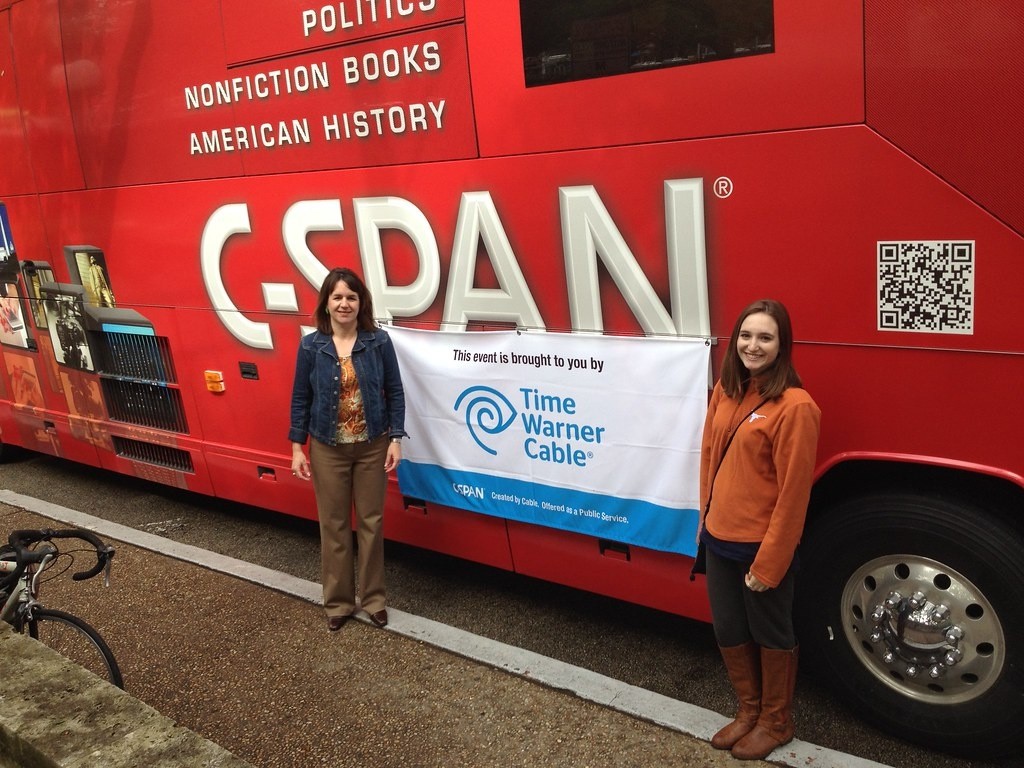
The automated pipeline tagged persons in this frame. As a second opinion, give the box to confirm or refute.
[286,267,411,630]
[696,299,821,761]
[0,284,20,334]
[89,256,115,308]
[56,301,86,369]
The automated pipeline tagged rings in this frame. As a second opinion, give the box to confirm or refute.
[747,583,752,588]
[293,472,295,476]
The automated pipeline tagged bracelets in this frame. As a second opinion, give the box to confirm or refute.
[391,437,402,443]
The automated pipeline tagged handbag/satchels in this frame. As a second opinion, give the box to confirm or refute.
[690,524,706,580]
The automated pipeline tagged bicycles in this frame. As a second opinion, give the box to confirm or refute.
[0,528,124,693]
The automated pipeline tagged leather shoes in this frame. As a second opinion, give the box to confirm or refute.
[370,608,388,627]
[329,614,351,629]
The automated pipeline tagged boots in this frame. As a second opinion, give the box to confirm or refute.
[731,638,800,759]
[711,638,760,749]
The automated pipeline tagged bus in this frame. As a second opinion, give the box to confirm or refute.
[0,0,1024,768]
